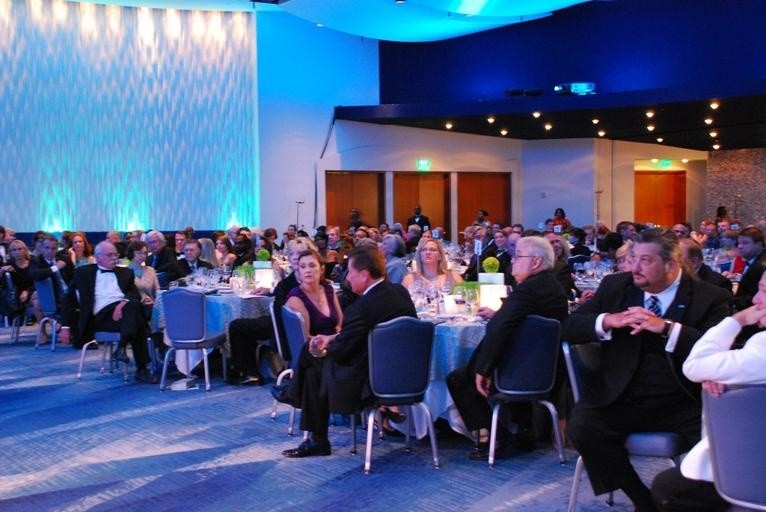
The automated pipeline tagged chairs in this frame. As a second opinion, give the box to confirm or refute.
[562,342,684,512]
[701,384,766,512]
[76,289,129,383]
[33,278,63,352]
[271,304,299,436]
[6,273,35,344]
[488,315,566,469]
[159,289,227,392]
[351,316,440,475]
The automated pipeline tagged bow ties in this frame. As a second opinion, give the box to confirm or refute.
[176,252,184,256]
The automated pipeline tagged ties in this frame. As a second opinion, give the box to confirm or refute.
[648,296,662,317]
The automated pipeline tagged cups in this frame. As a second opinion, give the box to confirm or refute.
[169,280,178,290]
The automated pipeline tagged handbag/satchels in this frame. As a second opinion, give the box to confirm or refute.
[0,289,24,317]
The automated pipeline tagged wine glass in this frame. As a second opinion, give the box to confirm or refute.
[572,259,614,284]
[442,241,464,271]
[701,247,728,263]
[408,280,479,322]
[186,265,246,295]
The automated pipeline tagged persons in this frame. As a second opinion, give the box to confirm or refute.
[2,205,766,512]
